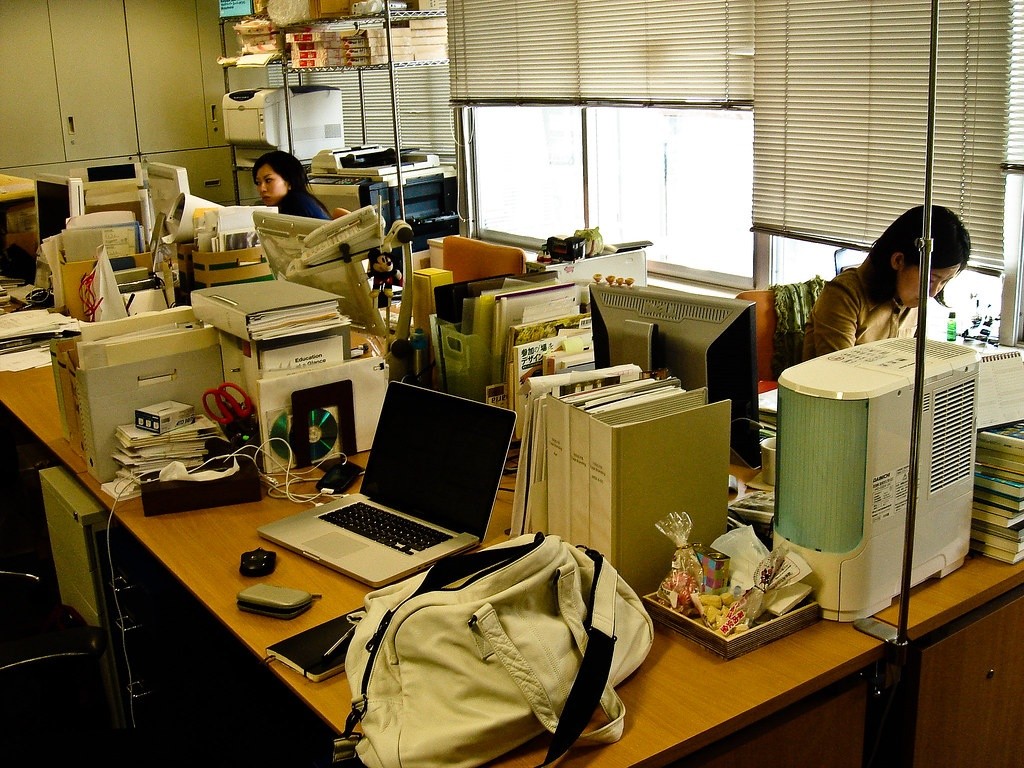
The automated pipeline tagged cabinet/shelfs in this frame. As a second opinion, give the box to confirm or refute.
[0,0,449,240]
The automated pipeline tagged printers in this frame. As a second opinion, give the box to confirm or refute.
[222,85,345,167]
[299,144,461,309]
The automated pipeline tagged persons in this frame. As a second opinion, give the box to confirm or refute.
[799,202,973,362]
[250,146,334,219]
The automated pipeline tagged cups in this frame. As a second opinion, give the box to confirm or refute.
[760,436,776,485]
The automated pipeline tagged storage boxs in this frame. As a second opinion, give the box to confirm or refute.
[219,0,254,18]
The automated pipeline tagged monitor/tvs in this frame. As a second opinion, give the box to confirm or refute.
[251,211,389,339]
[146,161,190,275]
[34,174,86,248]
[586,283,763,494]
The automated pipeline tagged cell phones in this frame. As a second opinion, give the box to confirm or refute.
[316,462,363,494]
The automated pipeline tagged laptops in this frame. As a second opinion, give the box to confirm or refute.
[257,380,517,589]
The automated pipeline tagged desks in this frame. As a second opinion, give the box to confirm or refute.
[0,264,1022,768]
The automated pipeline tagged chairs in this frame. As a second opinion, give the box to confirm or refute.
[0,567,137,768]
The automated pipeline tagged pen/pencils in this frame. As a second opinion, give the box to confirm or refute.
[322,624,357,656]
[126,293,135,309]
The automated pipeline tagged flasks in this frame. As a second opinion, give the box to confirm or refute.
[411,328,429,386]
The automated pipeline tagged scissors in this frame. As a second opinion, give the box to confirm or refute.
[202,383,252,424]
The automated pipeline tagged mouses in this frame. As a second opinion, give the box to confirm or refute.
[239,547,277,577]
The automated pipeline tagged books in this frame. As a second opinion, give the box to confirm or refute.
[439,265,732,599]
[46,207,387,495]
[969,421,1024,564]
[754,386,779,451]
[0,310,60,354]
[265,604,369,684]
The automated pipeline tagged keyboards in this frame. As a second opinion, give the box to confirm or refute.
[9,284,47,306]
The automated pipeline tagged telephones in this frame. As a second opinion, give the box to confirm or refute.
[301,195,389,265]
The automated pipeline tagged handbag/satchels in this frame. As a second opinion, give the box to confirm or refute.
[332,531,657,768]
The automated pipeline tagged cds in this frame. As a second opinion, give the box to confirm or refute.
[269,408,338,460]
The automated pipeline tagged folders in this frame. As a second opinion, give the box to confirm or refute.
[191,280,352,342]
[434,274,516,393]
[546,377,730,596]
